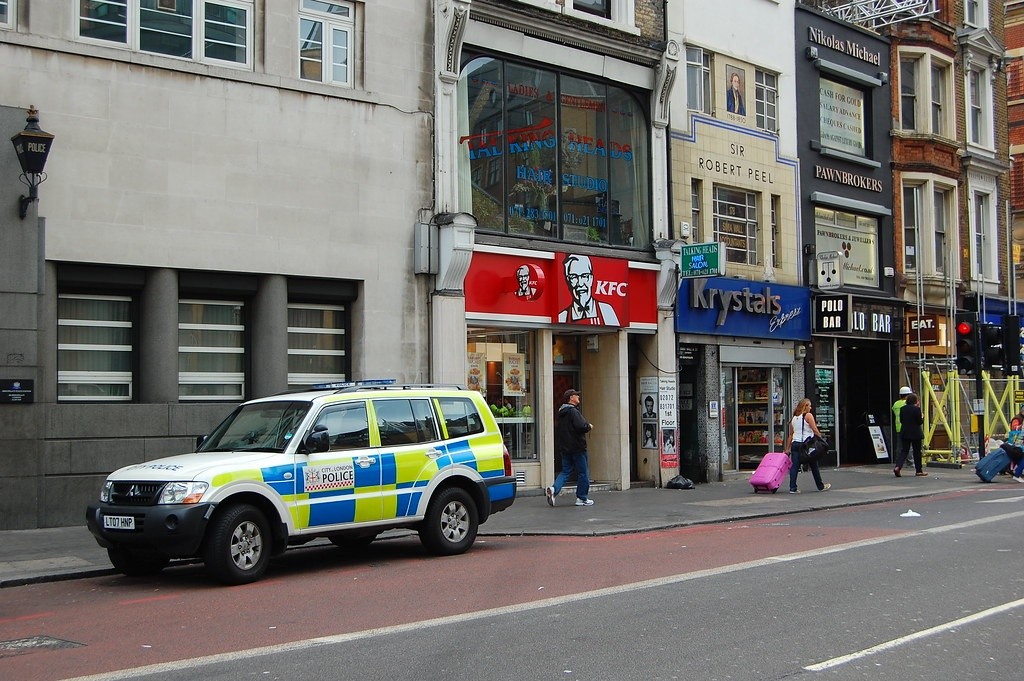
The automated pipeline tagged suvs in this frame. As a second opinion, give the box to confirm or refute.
[86,380,517,585]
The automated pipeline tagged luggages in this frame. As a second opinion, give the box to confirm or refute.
[749,451,793,494]
[975,443,1013,482]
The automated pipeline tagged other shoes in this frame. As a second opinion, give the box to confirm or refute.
[820,484,831,492]
[790,489,801,494]
[917,472,929,476]
[894,466,901,477]
[1012,475,1024,483]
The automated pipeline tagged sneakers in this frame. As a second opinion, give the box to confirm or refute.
[546,487,555,507]
[575,497,594,506]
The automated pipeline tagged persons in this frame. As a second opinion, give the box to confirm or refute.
[547,390,595,506]
[894,393,928,477]
[727,73,745,115]
[644,424,657,447]
[892,386,920,468]
[504,398,512,435]
[1012,404,1024,483]
[664,434,675,452]
[785,399,831,493]
[643,396,656,418]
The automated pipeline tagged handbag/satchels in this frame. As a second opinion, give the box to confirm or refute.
[798,435,830,464]
[1000,443,1022,464]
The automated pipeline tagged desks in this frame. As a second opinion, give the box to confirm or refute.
[495,417,535,457]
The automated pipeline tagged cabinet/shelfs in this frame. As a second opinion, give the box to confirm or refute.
[737,381,784,470]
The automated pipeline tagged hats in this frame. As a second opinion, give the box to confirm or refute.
[563,389,581,399]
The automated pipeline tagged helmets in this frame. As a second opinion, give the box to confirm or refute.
[899,386,912,394]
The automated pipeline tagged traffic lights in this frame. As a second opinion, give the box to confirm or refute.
[1001,315,1023,374]
[955,313,978,375]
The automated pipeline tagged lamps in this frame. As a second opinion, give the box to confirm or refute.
[10,105,55,220]
